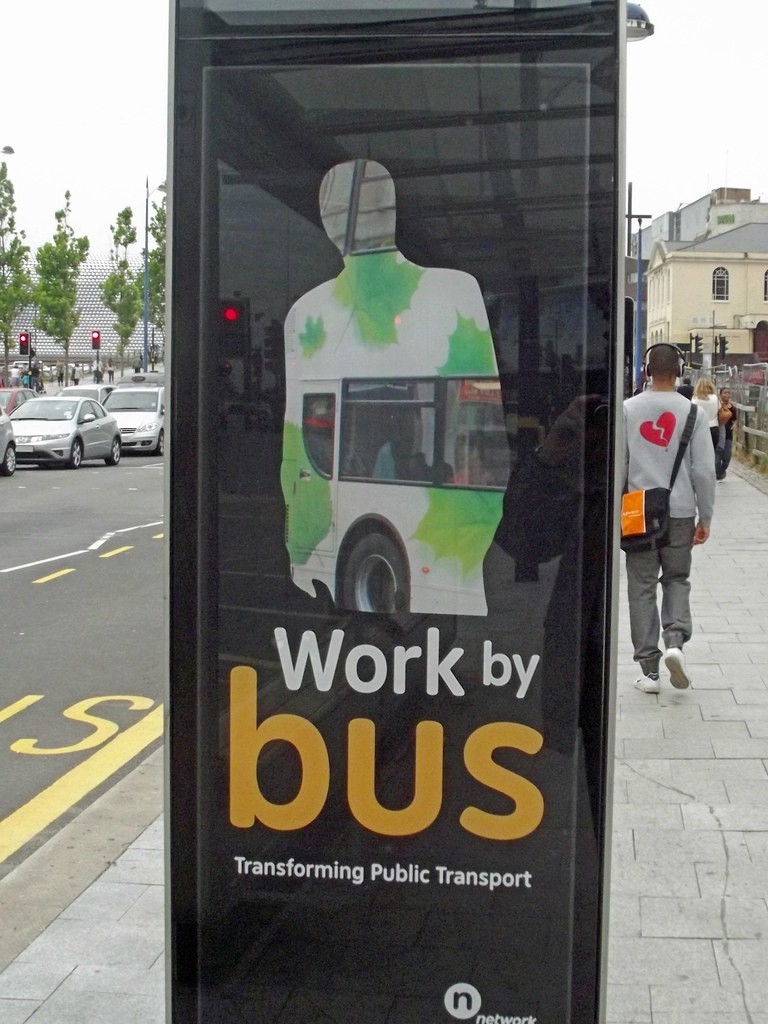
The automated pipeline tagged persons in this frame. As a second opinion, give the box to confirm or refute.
[57,364,64,386]
[131,355,143,373]
[622,343,715,696]
[713,387,739,484]
[675,375,723,448]
[72,363,84,386]
[91,357,105,383]
[21,364,40,395]
[105,358,115,385]
[303,412,497,487]
[490,383,612,859]
[0,375,5,389]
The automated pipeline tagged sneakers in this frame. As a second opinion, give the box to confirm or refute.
[633,677,660,693]
[664,648,689,689]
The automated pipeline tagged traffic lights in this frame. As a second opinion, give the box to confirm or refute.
[720,335,728,361]
[18,331,31,358]
[216,296,254,406]
[695,334,703,355]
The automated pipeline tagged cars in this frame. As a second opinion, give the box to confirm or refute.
[0,405,18,477]
[6,397,123,470]
[53,383,120,412]
[100,384,165,455]
[1,387,41,417]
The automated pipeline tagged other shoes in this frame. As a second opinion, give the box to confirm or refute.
[716,473,726,483]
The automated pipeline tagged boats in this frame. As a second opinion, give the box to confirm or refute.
[91,330,101,351]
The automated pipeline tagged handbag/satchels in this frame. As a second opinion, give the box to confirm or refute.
[620,487,670,552]
[717,394,732,426]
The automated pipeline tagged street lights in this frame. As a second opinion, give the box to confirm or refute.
[625,214,654,389]
[143,183,167,373]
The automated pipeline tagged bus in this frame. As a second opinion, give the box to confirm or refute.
[283,372,517,621]
